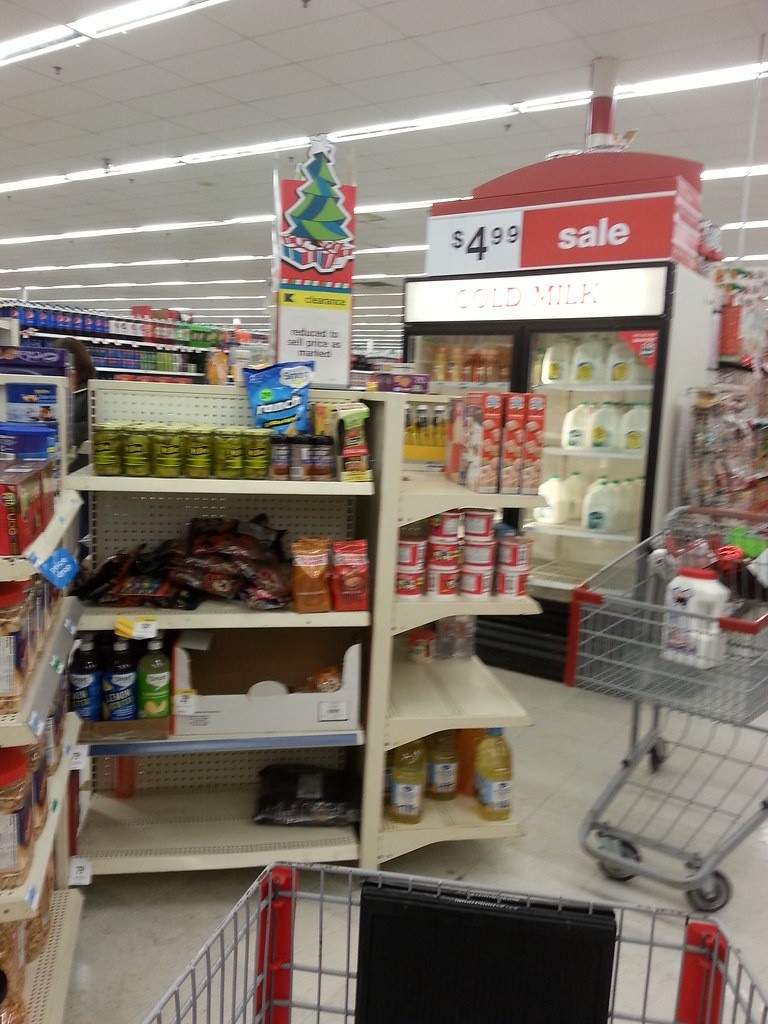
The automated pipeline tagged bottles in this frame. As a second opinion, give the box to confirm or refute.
[112,757,136,799]
[0,299,252,370]
[530,332,651,534]
[68,629,171,721]
[382,727,513,824]
[403,347,513,446]
[660,570,733,671]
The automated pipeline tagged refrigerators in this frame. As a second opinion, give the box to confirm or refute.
[403,259,722,692]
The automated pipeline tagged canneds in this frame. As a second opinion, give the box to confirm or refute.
[395,510,533,600]
[92,422,274,479]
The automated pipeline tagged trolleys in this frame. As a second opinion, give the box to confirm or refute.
[561,506,767,915]
[144,862,768,1024]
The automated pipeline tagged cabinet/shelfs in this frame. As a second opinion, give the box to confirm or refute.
[398,259,725,705]
[0,313,398,392]
[0,365,551,1023]
[713,353,768,605]
